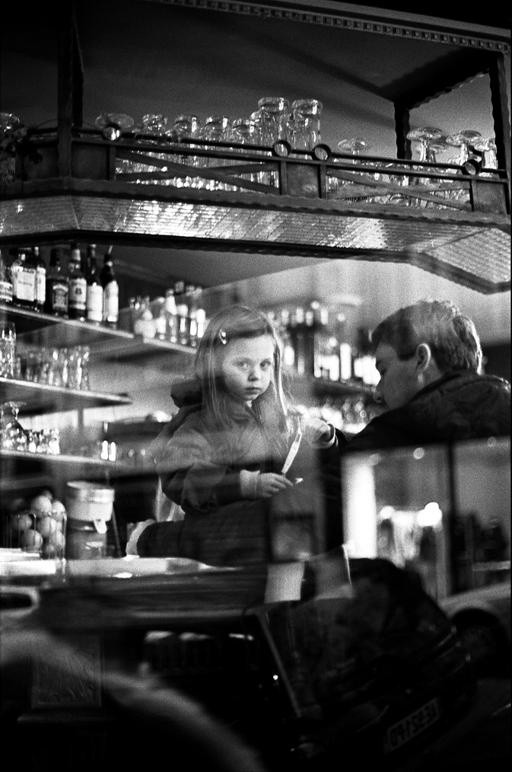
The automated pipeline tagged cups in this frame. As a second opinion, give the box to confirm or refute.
[0,324,91,454]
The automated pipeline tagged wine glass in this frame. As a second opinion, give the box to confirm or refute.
[0,96,497,214]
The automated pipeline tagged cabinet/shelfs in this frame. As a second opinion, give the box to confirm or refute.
[1,292,382,582]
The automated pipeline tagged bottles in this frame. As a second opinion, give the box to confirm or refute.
[1,241,207,349]
[270,303,352,382]
